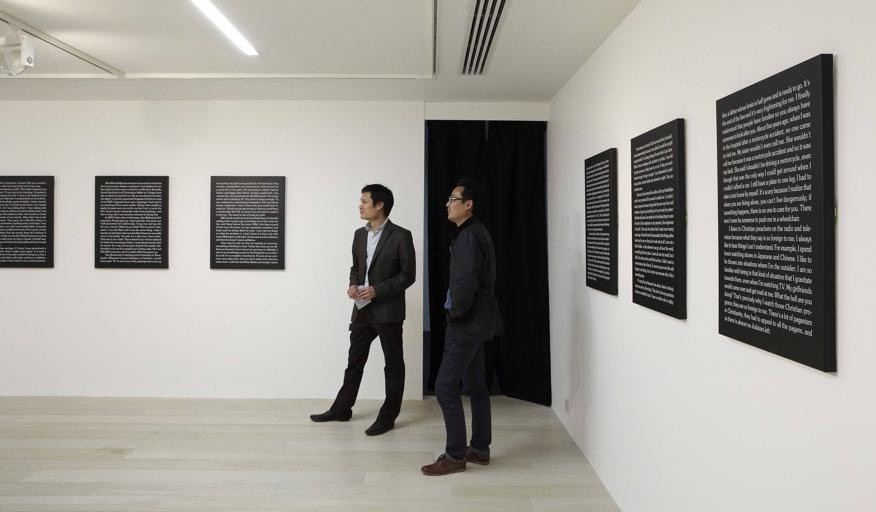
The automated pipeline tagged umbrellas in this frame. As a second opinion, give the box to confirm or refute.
[310,409,352,422]
[365,416,394,436]
[465,444,490,465]
[422,454,466,476]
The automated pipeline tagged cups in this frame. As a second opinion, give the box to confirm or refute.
[448,196,464,204]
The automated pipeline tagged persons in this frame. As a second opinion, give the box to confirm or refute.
[310,183,416,435]
[421,180,504,476]
